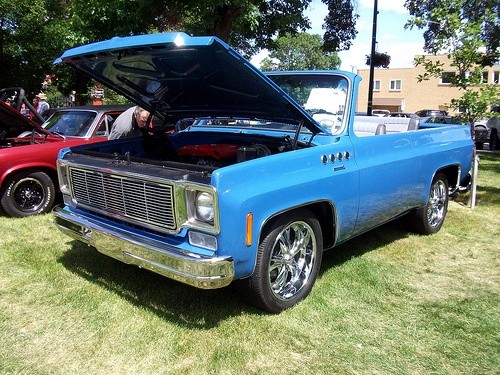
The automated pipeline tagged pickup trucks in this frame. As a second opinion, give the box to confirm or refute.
[49,31,481,314]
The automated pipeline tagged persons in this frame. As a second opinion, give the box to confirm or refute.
[108,105,154,141]
[4,96,50,120]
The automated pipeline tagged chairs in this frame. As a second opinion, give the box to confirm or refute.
[313,115,420,137]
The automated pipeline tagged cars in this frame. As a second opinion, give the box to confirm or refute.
[353,109,462,125]
[474,116,500,150]
[0,99,175,218]
